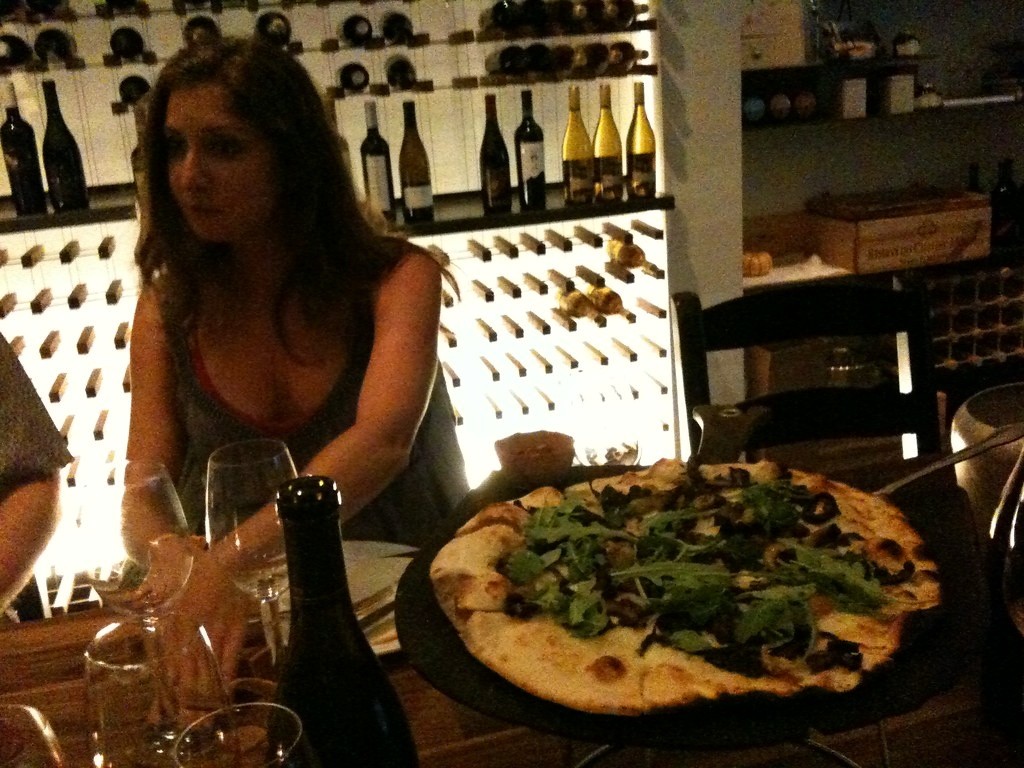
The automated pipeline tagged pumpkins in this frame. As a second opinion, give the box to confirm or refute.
[742,250,772,277]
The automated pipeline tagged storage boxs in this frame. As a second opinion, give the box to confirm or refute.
[809,185,994,272]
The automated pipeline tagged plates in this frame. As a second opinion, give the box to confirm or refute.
[278,540,422,655]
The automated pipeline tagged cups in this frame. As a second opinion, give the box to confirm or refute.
[0,608,313,768]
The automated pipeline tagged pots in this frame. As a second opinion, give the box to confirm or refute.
[391,403,958,746]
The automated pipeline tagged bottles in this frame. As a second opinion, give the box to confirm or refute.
[0,80,46,214]
[967,158,1021,247]
[985,40,1024,78]
[561,86,595,205]
[131,106,153,203]
[593,82,623,202]
[43,80,89,209]
[480,94,511,214]
[0,0,413,103]
[480,0,651,73]
[398,101,434,224]
[626,82,655,200]
[268,476,422,768]
[361,100,396,222]
[514,90,546,210]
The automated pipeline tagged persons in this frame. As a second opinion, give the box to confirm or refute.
[1,334,72,614]
[126,35,465,730]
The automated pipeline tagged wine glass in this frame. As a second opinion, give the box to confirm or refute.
[203,439,300,681]
[74,459,239,768]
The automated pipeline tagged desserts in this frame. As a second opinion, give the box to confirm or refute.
[494,431,575,486]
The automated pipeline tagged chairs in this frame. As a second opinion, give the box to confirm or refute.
[673,261,936,503]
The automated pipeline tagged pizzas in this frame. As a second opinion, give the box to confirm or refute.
[430,457,944,715]
